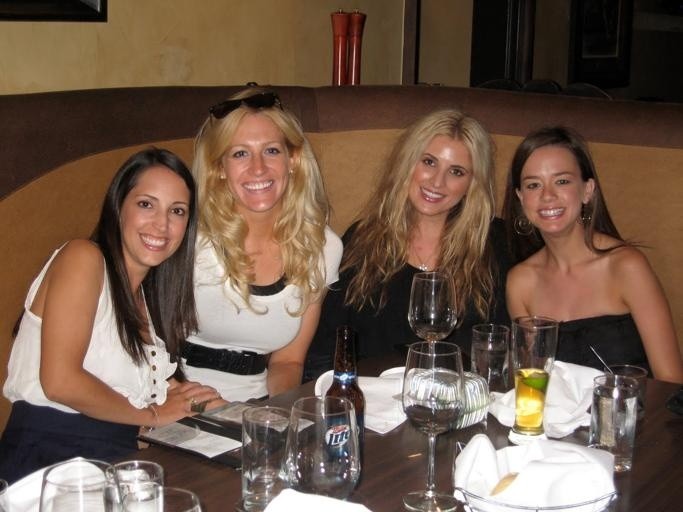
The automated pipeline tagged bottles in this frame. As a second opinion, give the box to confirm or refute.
[330,7,367,87]
[325,326,366,489]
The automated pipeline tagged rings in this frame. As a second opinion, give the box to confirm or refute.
[188,397,197,405]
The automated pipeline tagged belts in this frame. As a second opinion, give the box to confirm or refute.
[181,341,271,375]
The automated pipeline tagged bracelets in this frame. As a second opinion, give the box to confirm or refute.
[149,404,160,429]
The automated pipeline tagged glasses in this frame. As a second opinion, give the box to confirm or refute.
[208,92,282,126]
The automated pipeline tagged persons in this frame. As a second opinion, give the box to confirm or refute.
[0,145,230,492]
[166,87,344,402]
[500,124,683,386]
[302,108,538,384]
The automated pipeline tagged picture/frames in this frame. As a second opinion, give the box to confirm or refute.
[0,0,108,23]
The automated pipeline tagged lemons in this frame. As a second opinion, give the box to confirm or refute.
[521,375,547,391]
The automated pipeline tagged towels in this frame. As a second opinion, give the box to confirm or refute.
[492,358,605,437]
[263,485,371,512]
[311,366,407,435]
[1,458,108,510]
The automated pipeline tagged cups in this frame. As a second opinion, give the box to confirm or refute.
[238,404,295,511]
[589,373,639,475]
[600,364,649,417]
[465,323,511,384]
[506,314,559,445]
[283,394,359,500]
[37,456,202,512]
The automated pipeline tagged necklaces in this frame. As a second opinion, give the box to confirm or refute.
[411,243,441,271]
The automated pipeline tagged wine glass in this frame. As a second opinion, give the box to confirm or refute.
[398,341,464,512]
[403,269,461,369]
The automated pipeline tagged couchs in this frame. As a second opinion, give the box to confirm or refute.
[1,83,683,419]
